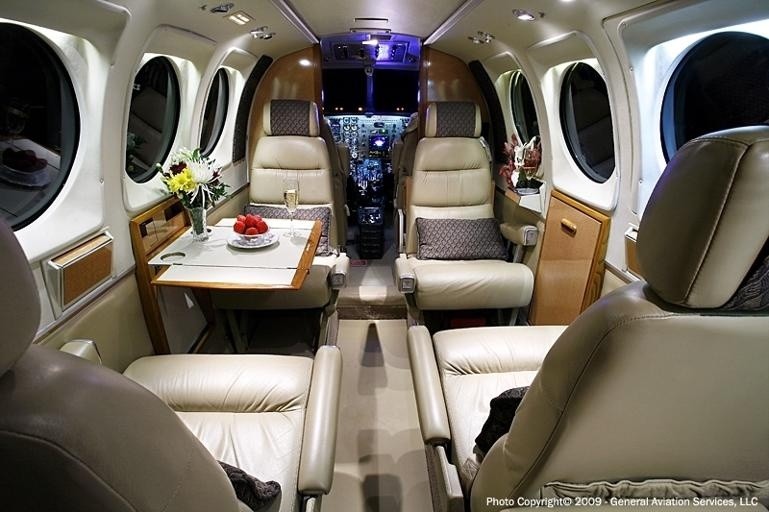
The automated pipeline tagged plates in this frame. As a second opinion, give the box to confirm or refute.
[227,233,280,249]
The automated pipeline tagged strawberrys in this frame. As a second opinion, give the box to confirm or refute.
[234,213,268,235]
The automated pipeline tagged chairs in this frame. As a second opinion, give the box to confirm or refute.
[2,216,343,511]
[390,111,421,216]
[408,118,769,511]
[391,98,537,329]
[323,118,353,213]
[195,97,350,352]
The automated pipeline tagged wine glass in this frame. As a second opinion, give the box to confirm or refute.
[282,179,302,238]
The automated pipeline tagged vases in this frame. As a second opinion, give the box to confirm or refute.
[185,204,210,240]
[511,180,541,197]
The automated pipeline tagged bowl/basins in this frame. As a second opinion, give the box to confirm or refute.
[233,228,272,245]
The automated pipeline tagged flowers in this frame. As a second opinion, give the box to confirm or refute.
[157,145,232,212]
[501,128,544,192]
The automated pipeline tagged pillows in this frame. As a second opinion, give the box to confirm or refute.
[471,385,536,456]
[204,460,283,509]
[413,216,510,261]
[241,198,331,256]
